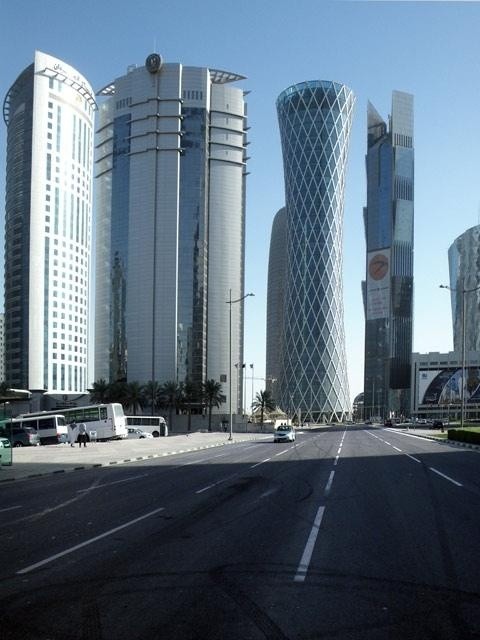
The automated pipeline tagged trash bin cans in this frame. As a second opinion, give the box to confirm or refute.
[0,438,12,466]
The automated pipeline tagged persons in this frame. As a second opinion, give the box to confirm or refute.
[222,416,229,431]
[448,378,457,403]
[77,420,87,447]
[67,419,78,447]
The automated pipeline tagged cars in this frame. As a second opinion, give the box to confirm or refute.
[12,427,41,447]
[274,426,295,443]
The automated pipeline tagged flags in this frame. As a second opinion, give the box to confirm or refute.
[241,364,246,369]
[234,363,238,368]
[249,364,253,369]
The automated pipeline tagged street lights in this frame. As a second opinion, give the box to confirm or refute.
[440,285,466,427]
[229,292,256,440]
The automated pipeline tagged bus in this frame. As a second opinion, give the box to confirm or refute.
[0,414,68,445]
[125,416,168,438]
[16,403,125,443]
